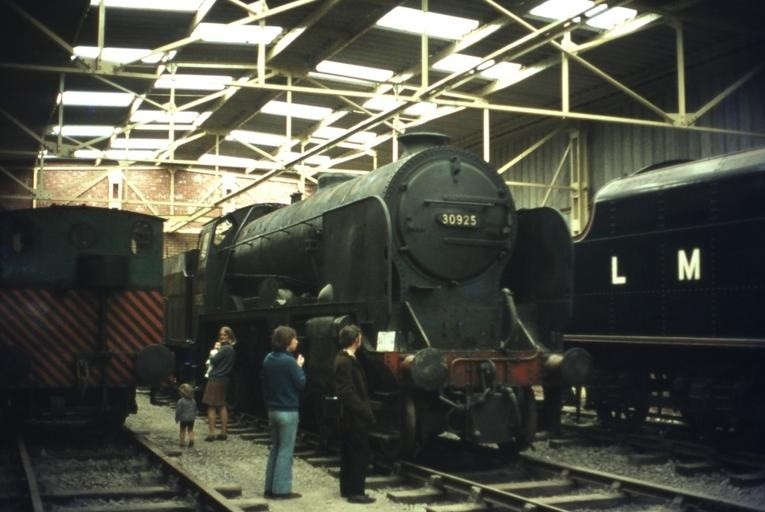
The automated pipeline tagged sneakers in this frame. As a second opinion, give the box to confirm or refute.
[178,438,195,449]
[262,489,304,500]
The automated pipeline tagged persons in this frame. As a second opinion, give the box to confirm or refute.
[174,383,198,449]
[262,325,307,502]
[332,324,377,504]
[204,341,221,379]
[201,326,237,442]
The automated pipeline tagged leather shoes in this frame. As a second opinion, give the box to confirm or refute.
[203,433,228,442]
[340,490,377,504]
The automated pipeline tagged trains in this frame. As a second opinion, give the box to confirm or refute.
[571,143,765,481]
[162,129,597,463]
[0,203,168,450]
